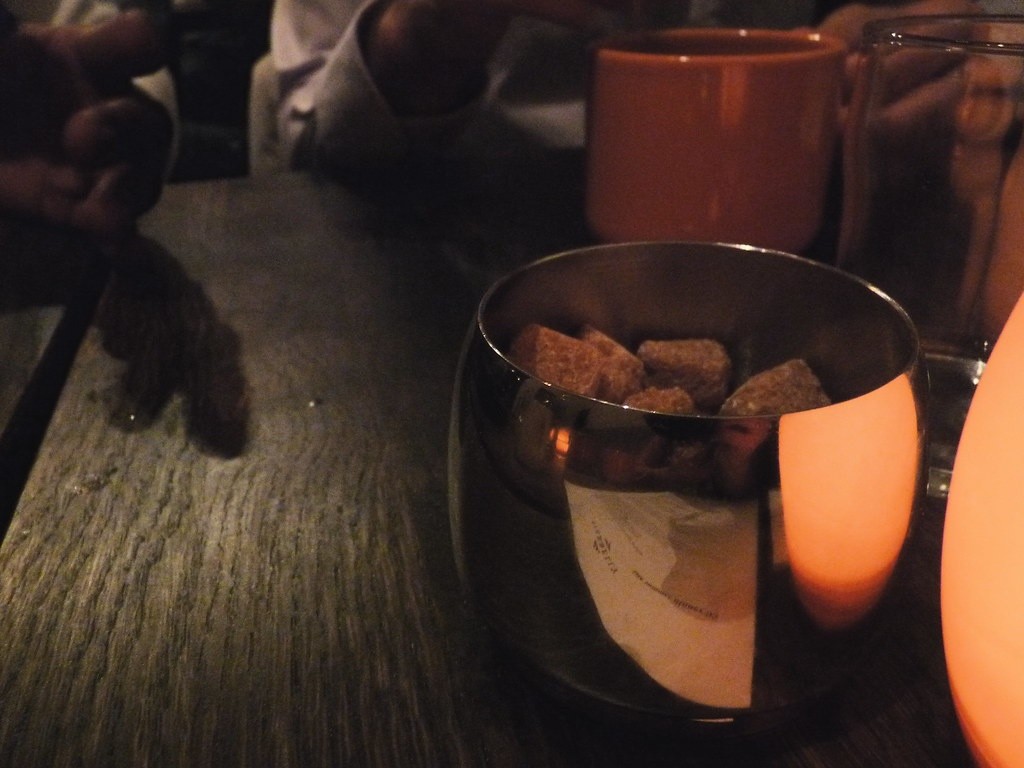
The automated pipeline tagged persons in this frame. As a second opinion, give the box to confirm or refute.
[0,0,183,239]
[268,0,1024,174]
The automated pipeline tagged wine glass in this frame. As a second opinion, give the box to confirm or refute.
[823,13,1023,501]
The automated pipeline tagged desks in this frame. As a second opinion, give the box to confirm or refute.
[0,159,973,768]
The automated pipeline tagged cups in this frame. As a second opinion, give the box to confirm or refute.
[585,28,849,256]
[448,245,933,733]
